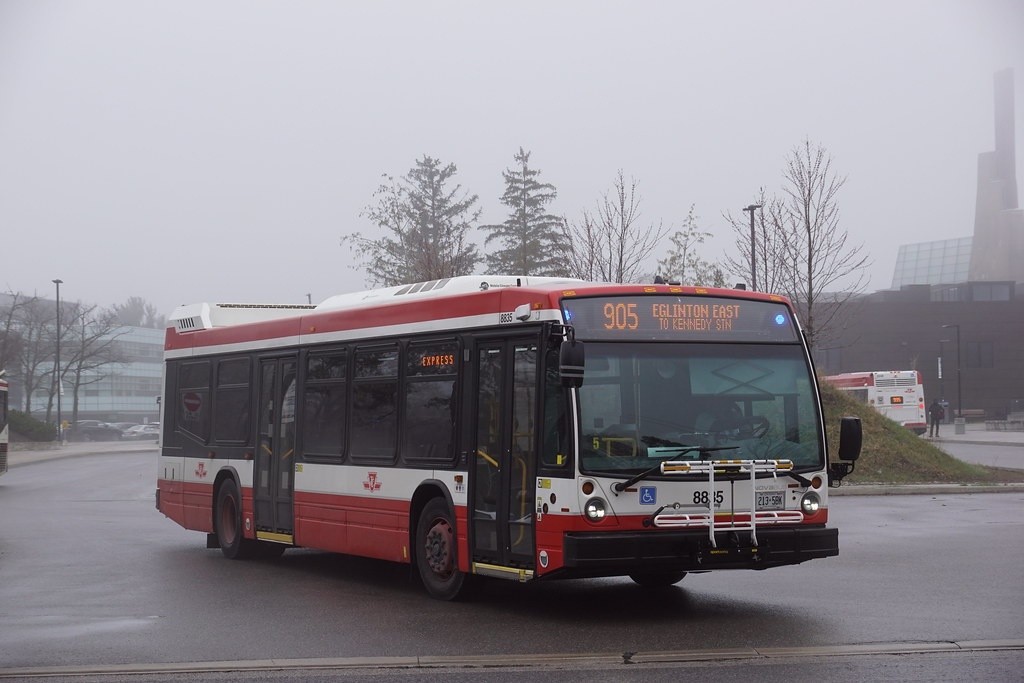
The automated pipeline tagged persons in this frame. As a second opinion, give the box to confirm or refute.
[928,398,945,437]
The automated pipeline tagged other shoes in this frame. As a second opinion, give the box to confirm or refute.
[929,436,933,437]
[936,435,939,437]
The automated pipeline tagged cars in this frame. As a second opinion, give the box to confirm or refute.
[76,419,160,440]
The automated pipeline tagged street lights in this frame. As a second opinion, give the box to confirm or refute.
[942,324,967,434]
[52,278,63,449]
[156,396,161,421]
[742,203,763,292]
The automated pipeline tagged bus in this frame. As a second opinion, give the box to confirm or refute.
[817,371,928,438]
[155,276,863,603]
